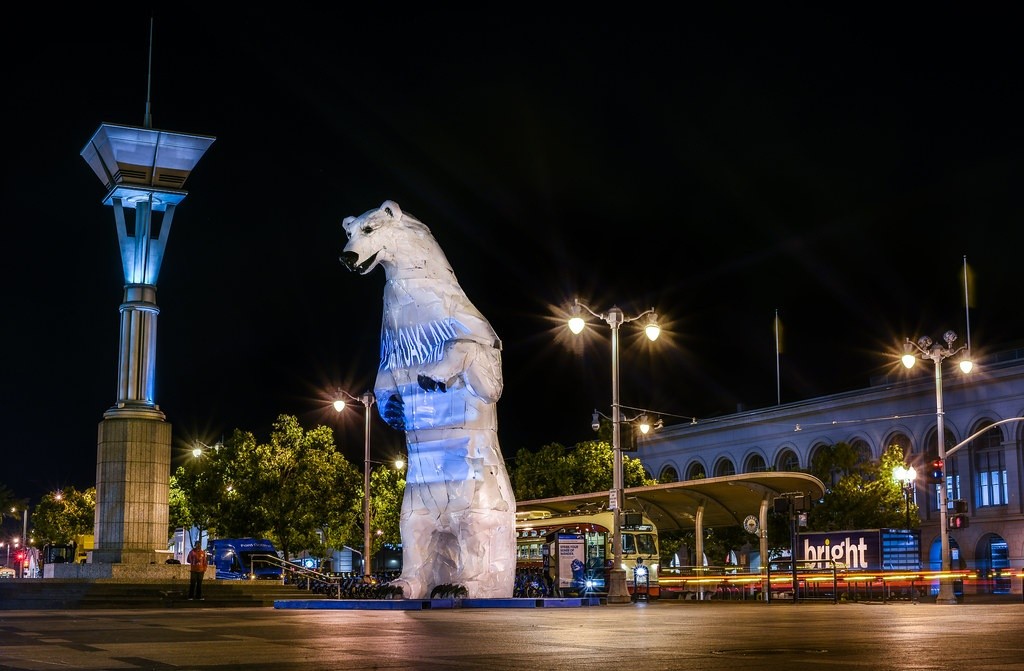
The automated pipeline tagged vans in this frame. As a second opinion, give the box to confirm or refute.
[204,537,284,583]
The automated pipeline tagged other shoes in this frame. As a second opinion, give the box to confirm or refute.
[188,597,193,601]
[196,598,205,600]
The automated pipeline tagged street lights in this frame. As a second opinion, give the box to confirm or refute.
[334,387,374,577]
[901,330,974,604]
[193,439,219,458]
[895,464,917,530]
[567,297,661,604]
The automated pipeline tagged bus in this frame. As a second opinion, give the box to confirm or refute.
[515,511,661,589]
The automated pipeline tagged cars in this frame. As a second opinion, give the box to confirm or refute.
[372,545,402,580]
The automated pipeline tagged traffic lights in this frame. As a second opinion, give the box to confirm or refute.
[954,498,970,513]
[948,514,969,529]
[929,458,944,480]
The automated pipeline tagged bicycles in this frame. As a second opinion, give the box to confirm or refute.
[294,574,551,601]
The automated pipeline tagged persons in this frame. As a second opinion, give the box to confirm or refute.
[570,559,583,586]
[186,541,207,600]
